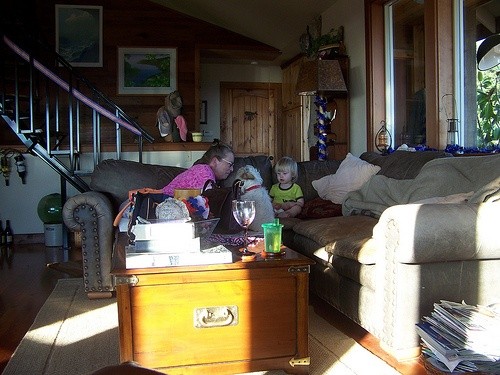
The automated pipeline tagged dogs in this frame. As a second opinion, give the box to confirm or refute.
[235,164,276,233]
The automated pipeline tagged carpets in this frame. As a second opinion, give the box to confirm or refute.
[2,279,403,375]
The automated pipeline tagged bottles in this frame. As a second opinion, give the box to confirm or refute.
[0,220,4,244]
[4,220,14,243]
[1,157,11,186]
[261,219,284,252]
[15,156,26,185]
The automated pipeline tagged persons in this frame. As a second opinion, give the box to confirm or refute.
[269,156,304,222]
[162,139,235,197]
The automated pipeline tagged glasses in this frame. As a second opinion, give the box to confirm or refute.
[216,155,235,169]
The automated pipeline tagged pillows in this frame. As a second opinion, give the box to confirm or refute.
[312,153,382,204]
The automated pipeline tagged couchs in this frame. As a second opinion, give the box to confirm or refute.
[62,152,500,356]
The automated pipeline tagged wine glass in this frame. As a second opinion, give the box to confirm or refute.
[231,199,256,255]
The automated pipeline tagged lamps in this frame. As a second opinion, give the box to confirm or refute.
[476,35,500,71]
[296,60,347,161]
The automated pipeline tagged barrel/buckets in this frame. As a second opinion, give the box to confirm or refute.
[44,224,62,247]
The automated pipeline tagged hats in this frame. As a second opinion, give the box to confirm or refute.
[155,107,171,137]
[174,115,187,142]
[165,90,182,118]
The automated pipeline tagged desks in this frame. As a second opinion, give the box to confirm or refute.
[111,232,316,375]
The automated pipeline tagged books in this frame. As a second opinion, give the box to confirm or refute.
[125,222,233,269]
[415,300,500,374]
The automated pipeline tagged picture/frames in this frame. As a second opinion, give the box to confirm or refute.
[200,100,208,125]
[53,4,105,69]
[116,44,178,97]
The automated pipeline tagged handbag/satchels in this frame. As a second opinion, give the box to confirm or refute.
[201,179,243,235]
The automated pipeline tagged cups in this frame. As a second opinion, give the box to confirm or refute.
[191,132,203,143]
